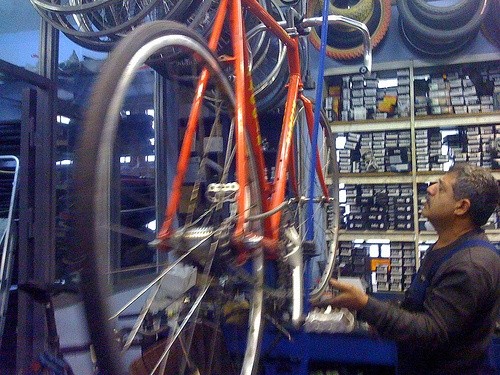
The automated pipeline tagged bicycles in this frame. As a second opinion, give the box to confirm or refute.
[77,2,342,375]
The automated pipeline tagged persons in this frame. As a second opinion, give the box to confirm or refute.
[312,161,499,375]
